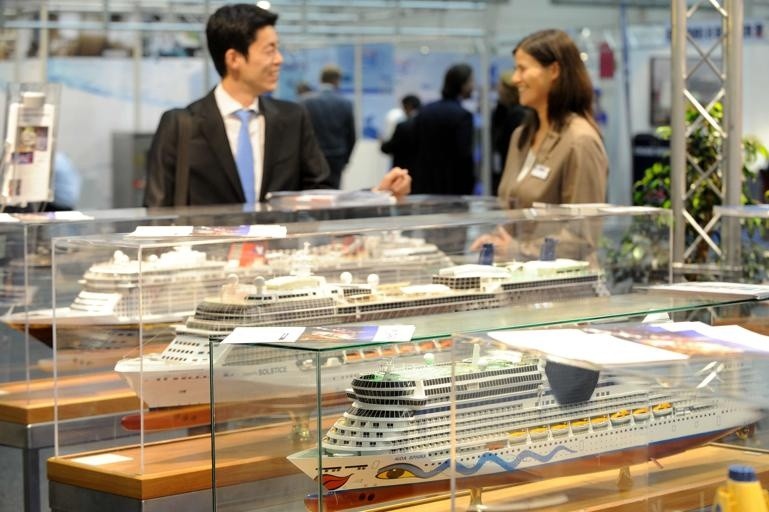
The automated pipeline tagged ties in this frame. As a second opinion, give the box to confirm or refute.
[232,109,255,203]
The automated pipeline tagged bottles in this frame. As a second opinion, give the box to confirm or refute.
[713,465,769,512]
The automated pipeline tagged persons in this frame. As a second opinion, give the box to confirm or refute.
[40,154,80,214]
[143,3,412,209]
[469,28,609,271]
[294,62,529,197]
